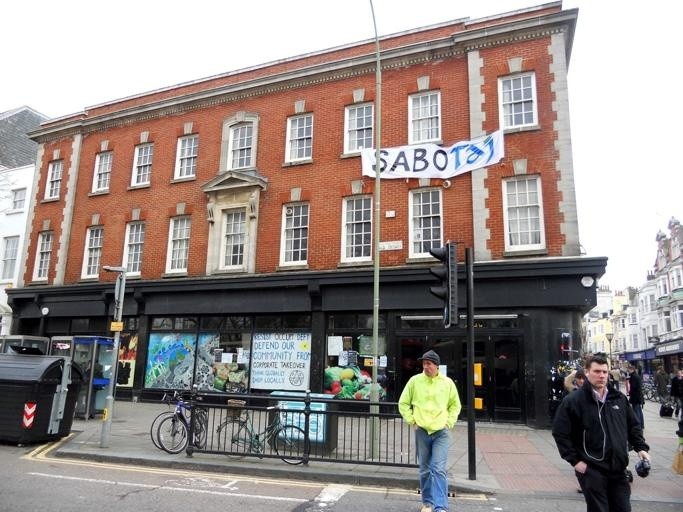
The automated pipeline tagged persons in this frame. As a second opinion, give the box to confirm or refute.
[561,370,588,494]
[552,354,650,511]
[398,349,462,512]
[610,361,683,476]
[86,357,102,419]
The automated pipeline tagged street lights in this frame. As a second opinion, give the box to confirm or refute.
[605,332,614,369]
[99,263,127,444]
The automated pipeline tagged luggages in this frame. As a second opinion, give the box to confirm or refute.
[660,404,674,417]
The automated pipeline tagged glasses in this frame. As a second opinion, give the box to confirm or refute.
[575,374,585,379]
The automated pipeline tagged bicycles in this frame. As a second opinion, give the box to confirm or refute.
[147,391,205,450]
[214,401,311,465]
[158,389,206,454]
[641,379,670,401]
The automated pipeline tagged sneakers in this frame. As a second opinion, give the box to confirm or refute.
[421,505,446,512]
[577,489,582,493]
[674,412,679,418]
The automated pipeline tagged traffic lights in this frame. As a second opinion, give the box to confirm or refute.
[426,237,459,330]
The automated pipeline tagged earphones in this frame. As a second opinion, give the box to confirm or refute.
[585,373,589,377]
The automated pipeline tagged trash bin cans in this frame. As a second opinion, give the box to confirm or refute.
[0,345,84,447]
[267,391,338,455]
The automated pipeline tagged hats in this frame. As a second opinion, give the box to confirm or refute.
[626,365,635,369]
[418,350,441,365]
[576,364,585,376]
